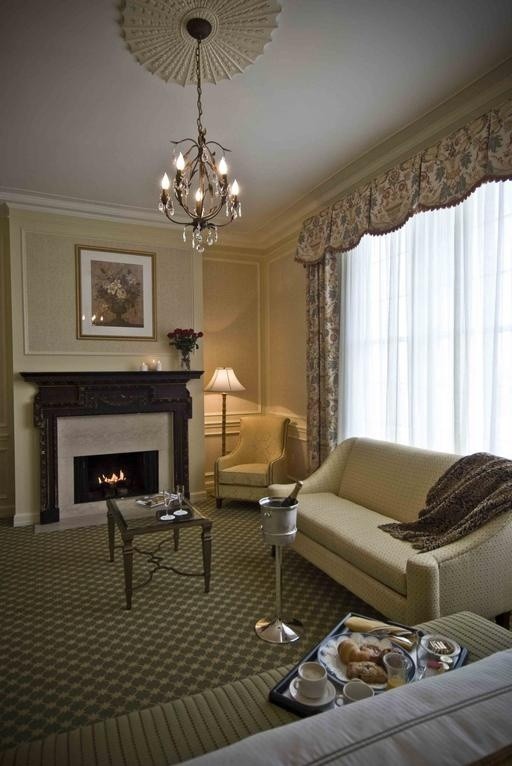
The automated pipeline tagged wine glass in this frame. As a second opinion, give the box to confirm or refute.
[175,483,186,514]
[161,488,172,517]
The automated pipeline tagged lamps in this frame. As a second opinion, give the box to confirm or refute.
[159,18,242,253]
[204,367,246,456]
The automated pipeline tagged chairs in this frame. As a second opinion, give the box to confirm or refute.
[214,415,290,509]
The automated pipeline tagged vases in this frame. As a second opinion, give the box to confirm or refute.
[178,350,191,371]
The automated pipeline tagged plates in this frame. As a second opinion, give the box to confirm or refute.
[317,633,417,690]
[421,632,461,659]
[365,627,419,653]
[288,678,336,708]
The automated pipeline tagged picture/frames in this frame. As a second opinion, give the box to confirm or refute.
[75,244,156,341]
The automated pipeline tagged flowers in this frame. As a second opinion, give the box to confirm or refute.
[168,328,203,368]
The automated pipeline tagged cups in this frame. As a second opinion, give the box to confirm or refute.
[335,681,374,707]
[292,661,331,703]
[382,652,412,690]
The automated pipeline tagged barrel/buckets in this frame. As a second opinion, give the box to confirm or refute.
[259,496,300,545]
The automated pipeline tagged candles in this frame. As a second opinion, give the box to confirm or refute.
[142,360,162,371]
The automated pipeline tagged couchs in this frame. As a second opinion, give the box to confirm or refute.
[0,611,512,766]
[267,437,512,625]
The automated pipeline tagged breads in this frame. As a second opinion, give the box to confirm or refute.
[338,641,403,683]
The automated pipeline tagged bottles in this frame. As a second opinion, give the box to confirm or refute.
[280,480,303,506]
